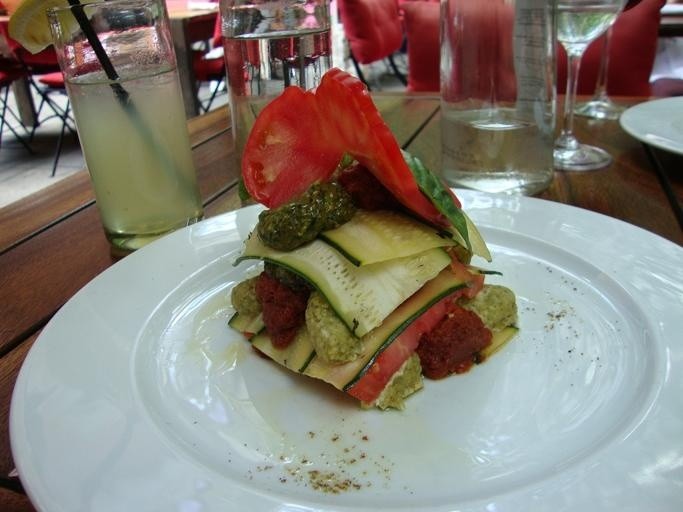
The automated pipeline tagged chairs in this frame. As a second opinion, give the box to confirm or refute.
[1,15,408,177]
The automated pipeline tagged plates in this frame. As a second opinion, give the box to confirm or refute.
[622,97,682,158]
[6,186,681,512]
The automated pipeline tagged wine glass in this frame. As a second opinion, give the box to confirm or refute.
[541,2,624,172]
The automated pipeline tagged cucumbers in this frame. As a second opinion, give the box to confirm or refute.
[221,147,502,393]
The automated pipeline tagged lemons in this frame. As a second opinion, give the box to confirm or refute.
[7,0,104,55]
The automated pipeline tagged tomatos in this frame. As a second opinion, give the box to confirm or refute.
[240,70,463,229]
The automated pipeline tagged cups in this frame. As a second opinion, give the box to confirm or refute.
[217,0,334,205]
[435,2,555,195]
[46,1,207,249]
